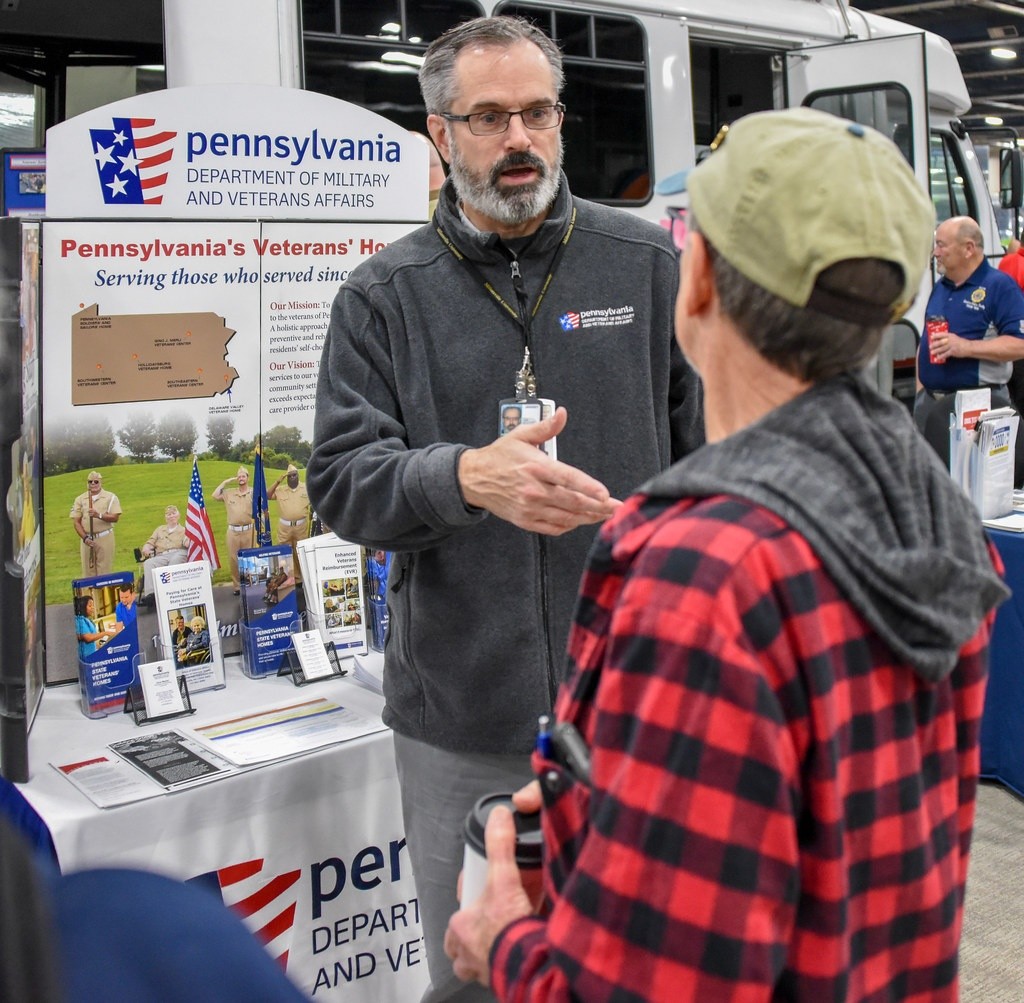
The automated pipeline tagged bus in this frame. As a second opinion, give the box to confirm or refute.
[161,3,1023,404]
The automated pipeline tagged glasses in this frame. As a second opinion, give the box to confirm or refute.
[88,479,99,484]
[666,204,691,248]
[504,416,521,420]
[435,103,569,135]
[287,474,298,478]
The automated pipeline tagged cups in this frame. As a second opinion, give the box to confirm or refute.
[459,791,546,915]
[925,314,949,364]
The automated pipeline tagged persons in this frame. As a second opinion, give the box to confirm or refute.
[307,14,706,1003]
[440,104,1023,1003]
[19,450,392,672]
[998,230,1024,416]
[911,216,1024,471]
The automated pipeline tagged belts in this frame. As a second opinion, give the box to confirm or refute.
[86,529,113,539]
[923,384,1007,399]
[155,548,185,556]
[228,523,255,531]
[280,518,306,526]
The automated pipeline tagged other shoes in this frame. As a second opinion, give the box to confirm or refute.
[264,597,278,605]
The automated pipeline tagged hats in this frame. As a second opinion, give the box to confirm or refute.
[658,108,932,323]
[237,466,249,477]
[287,464,298,473]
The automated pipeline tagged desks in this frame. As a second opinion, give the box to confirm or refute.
[978,491,1024,798]
[27,629,431,1003]
